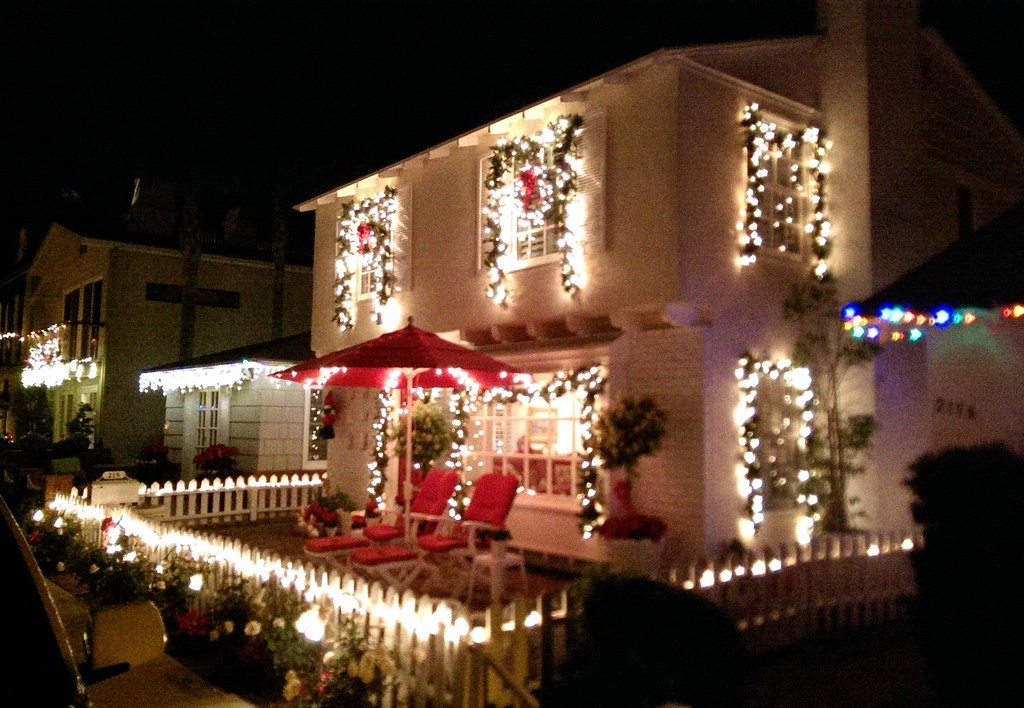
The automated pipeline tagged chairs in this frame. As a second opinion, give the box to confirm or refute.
[302,470,458,574]
[350,474,518,605]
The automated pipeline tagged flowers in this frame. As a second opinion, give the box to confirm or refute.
[353,517,364,529]
[366,502,378,518]
[194,443,240,465]
[325,513,338,529]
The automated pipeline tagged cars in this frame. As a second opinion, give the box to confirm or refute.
[0,493,170,708]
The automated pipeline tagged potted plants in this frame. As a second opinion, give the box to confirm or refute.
[478,525,514,556]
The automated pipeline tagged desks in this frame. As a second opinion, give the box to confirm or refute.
[465,554,526,608]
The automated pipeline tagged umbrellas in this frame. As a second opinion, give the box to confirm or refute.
[268,315,532,548]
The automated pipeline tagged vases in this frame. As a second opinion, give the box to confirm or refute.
[326,528,336,537]
[365,518,378,527]
[352,530,363,536]
